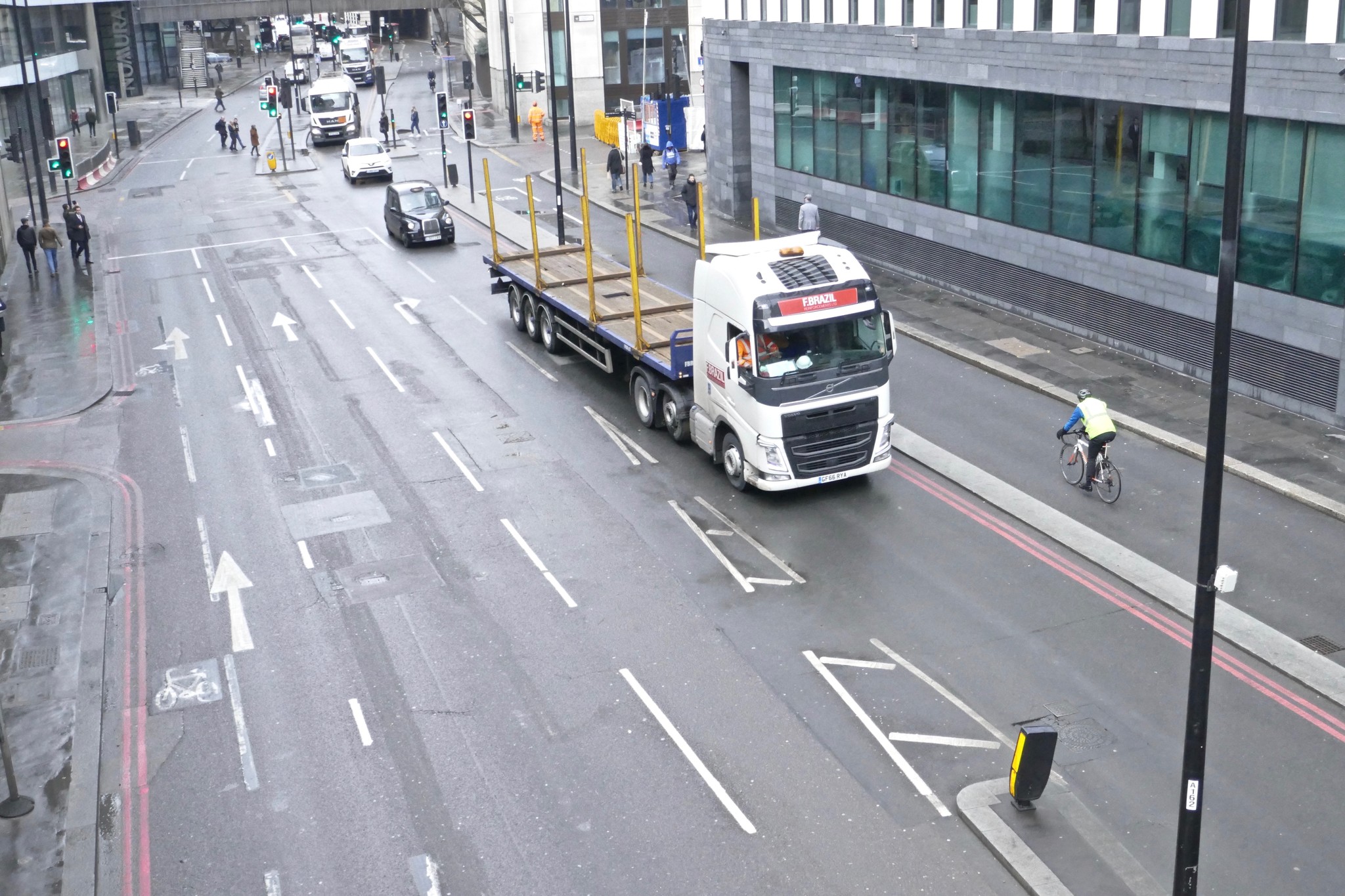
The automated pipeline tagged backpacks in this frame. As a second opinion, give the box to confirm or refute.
[215,121,224,131]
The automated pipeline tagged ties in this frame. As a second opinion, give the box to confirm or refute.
[79,216,82,222]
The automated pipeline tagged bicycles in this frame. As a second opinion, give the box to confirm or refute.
[1059,427,1123,503]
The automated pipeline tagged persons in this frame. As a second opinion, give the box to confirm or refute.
[85,108,97,138]
[1057,389,1116,491]
[662,141,680,189]
[182,15,438,93]
[380,105,421,145]
[528,102,545,142]
[69,109,81,136]
[798,194,820,231]
[733,334,780,369]
[38,219,64,277]
[62,202,82,256]
[606,144,625,193]
[681,174,696,228]
[214,85,226,112]
[700,124,706,154]
[218,116,262,156]
[17,218,39,278]
[70,206,94,263]
[639,142,655,189]
[851,74,1142,160]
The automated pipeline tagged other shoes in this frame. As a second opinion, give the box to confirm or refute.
[1103,462,1109,469]
[619,186,623,191]
[409,134,421,138]
[643,184,647,187]
[650,182,653,188]
[29,269,39,278]
[383,142,389,144]
[689,224,698,232]
[72,253,82,259]
[670,185,675,189]
[220,144,261,157]
[541,139,544,141]
[612,190,617,192]
[51,269,59,278]
[533,140,537,142]
[1078,483,1092,492]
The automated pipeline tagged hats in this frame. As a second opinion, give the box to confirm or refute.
[532,101,537,106]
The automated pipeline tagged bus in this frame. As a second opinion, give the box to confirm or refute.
[290,24,314,57]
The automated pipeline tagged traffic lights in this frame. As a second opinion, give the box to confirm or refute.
[462,109,477,141]
[516,73,531,89]
[391,114,394,124]
[105,92,119,115]
[261,85,279,118]
[437,92,450,128]
[3,133,21,163]
[429,79,436,93]
[264,77,272,86]
[256,41,261,51]
[387,28,393,39]
[47,137,75,180]
[535,70,546,92]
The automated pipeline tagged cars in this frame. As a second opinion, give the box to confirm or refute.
[277,34,291,48]
[313,37,336,58]
[341,137,393,184]
[206,52,232,63]
[384,180,455,248]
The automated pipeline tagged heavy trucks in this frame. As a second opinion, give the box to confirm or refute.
[483,148,897,493]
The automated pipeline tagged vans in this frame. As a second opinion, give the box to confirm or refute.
[284,59,309,83]
[304,20,342,42]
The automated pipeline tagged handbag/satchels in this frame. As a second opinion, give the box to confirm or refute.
[379,127,386,133]
[621,164,626,175]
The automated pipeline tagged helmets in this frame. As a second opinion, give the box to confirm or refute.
[1077,389,1092,400]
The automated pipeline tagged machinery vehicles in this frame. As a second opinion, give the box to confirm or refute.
[301,71,361,144]
[344,25,370,41]
[336,36,376,87]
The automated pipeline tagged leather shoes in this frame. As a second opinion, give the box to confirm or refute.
[85,260,94,264]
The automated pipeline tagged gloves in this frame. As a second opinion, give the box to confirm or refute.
[1080,425,1088,432]
[638,162,642,165]
[1056,426,1068,439]
